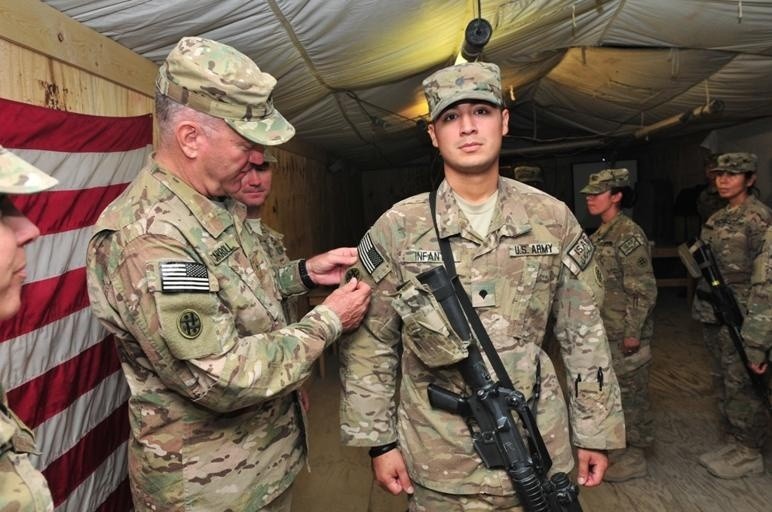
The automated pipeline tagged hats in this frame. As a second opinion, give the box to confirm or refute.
[581,168,634,194]
[710,153,758,176]
[154,37,296,164]
[0,144,60,195]
[515,165,541,183]
[421,61,502,122]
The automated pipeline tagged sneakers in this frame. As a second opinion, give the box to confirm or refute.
[698,436,764,479]
[605,445,646,483]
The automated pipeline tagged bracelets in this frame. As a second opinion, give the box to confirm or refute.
[299,260,317,289]
[366,442,394,458]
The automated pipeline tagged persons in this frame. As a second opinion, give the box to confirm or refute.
[581,168,658,482]
[232,149,310,411]
[339,61,627,512]
[0,145,60,512]
[697,154,729,219]
[691,152,772,480]
[514,166,544,185]
[85,36,370,512]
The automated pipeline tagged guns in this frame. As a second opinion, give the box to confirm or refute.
[684,229,769,399]
[394,265,587,511]
